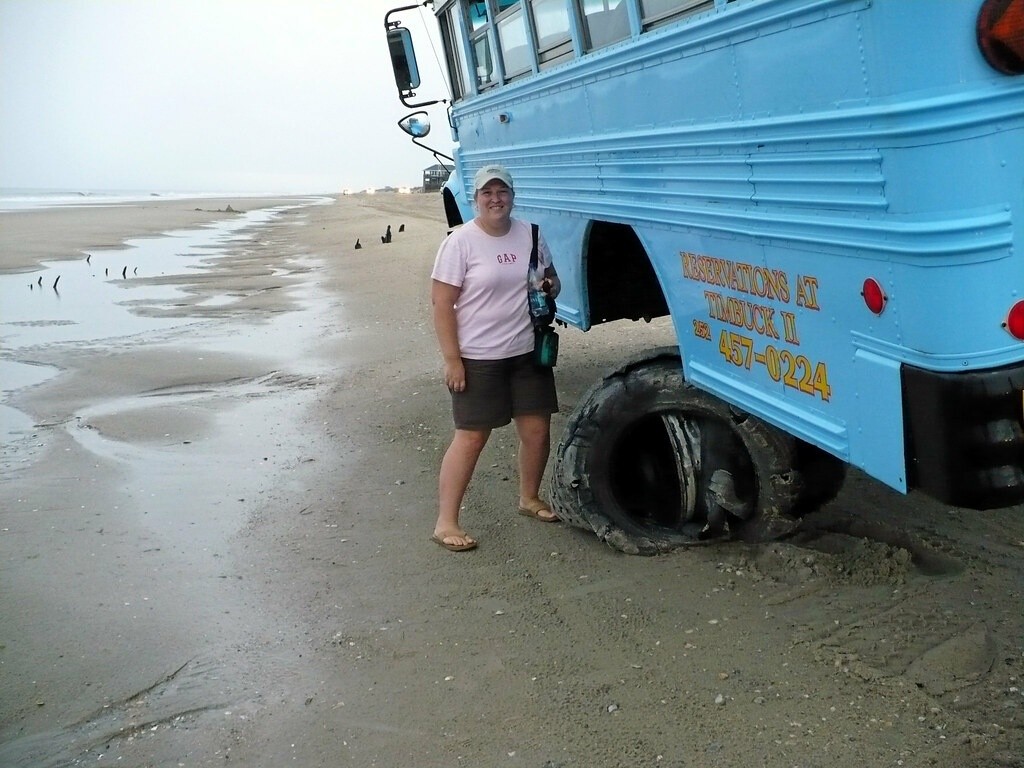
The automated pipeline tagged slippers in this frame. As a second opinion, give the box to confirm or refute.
[430,529,477,552]
[519,502,558,522]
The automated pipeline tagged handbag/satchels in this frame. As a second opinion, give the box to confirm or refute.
[526,221,557,326]
[537,327,559,366]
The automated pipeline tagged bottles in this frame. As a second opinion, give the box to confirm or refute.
[527,263,549,316]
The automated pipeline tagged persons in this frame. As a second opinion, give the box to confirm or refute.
[429,166,561,546]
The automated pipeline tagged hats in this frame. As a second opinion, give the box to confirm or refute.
[475,164,513,189]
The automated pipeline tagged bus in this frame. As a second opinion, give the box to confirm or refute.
[379,1,1024,556]
